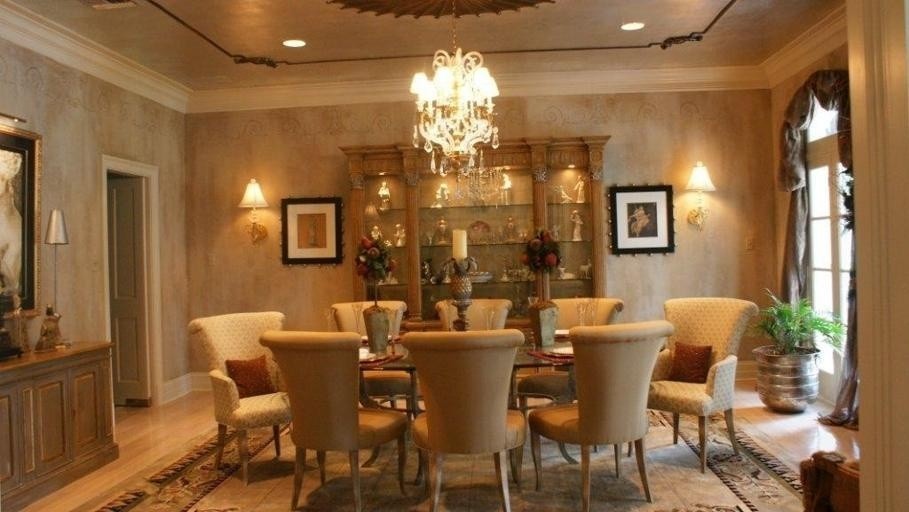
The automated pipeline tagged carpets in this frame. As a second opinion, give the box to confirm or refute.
[71,398,806,511]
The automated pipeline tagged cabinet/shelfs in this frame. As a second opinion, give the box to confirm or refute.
[0,340,119,511]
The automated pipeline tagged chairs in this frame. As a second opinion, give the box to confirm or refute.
[513,296,624,482]
[528,320,675,511]
[329,301,409,345]
[400,328,527,512]
[259,329,409,512]
[187,311,292,488]
[649,297,759,472]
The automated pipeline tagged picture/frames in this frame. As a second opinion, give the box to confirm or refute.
[609,185,674,255]
[281,197,342,264]
[0,123,43,323]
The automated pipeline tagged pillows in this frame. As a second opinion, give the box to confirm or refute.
[225,354,277,399]
[669,343,712,384]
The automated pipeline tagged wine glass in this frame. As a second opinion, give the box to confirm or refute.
[436,215,448,244]
[577,296,599,326]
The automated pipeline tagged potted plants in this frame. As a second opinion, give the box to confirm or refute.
[738,285,846,414]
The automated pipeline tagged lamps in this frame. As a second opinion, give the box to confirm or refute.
[45,209,68,313]
[409,0,501,157]
[685,161,716,226]
[237,179,269,244]
